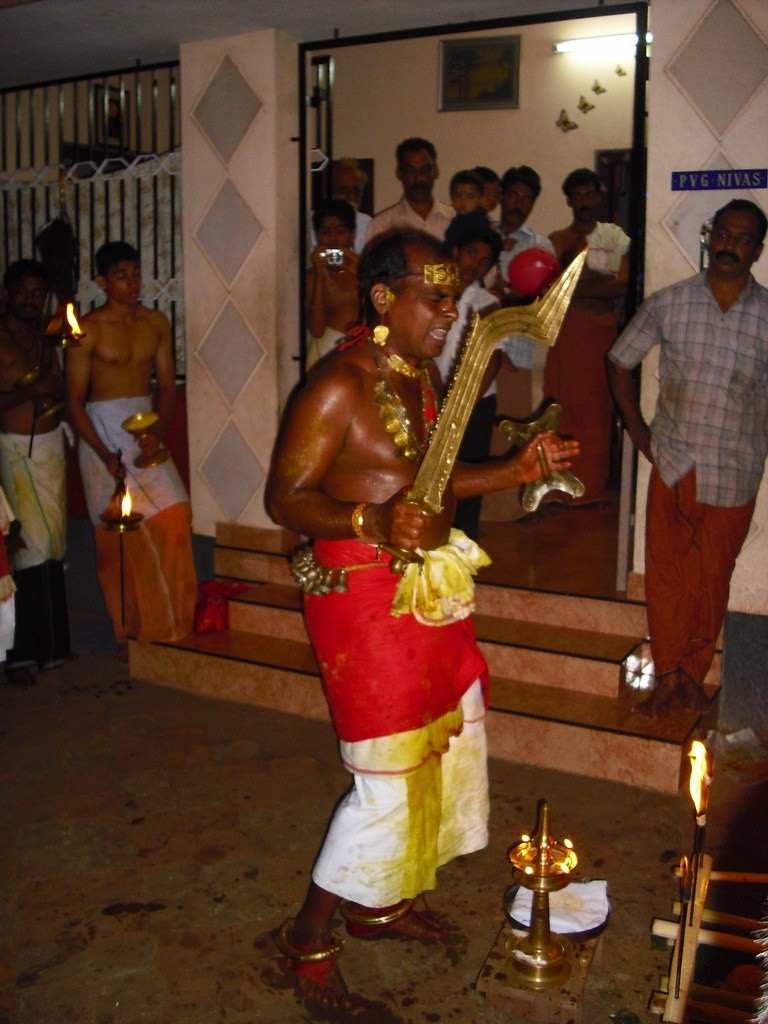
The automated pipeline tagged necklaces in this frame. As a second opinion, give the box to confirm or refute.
[339,323,441,461]
[373,336,428,380]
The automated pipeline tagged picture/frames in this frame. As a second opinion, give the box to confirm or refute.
[437,34,521,111]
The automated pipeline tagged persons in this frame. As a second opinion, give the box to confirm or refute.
[305,139,630,544]
[0,258,78,685]
[605,200,768,722]
[65,241,198,652]
[272,228,580,1010]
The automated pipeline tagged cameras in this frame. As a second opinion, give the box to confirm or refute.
[326,249,345,264]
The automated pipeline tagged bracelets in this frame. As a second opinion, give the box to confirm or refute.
[351,500,374,542]
